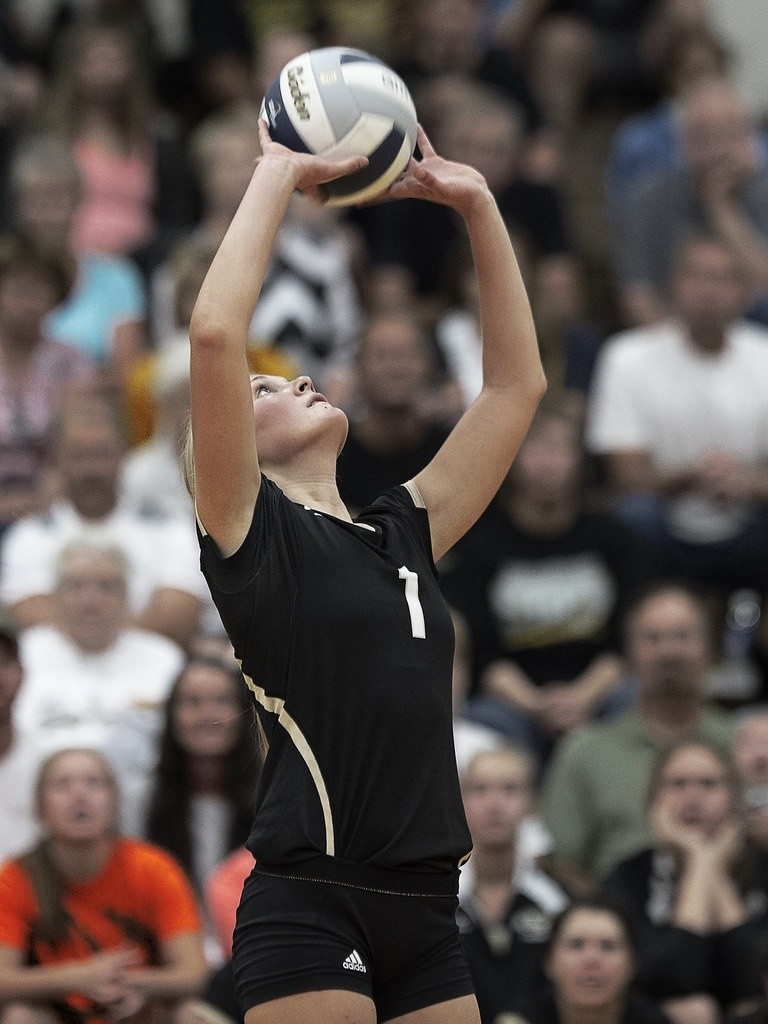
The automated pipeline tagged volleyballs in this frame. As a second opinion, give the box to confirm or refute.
[259,46,419,209]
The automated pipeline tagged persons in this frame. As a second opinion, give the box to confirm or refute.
[1,1,768,1024]
[182,114,552,1024]
